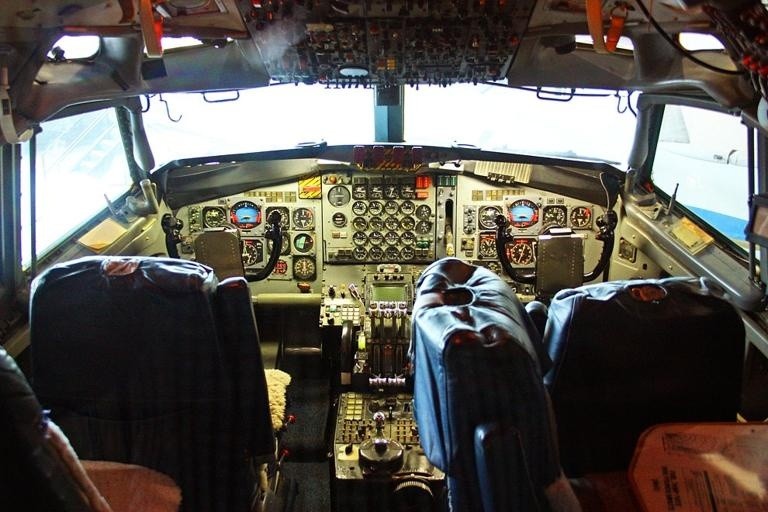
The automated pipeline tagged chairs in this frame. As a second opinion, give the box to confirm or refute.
[27,256,289,509]
[532,278,744,491]
